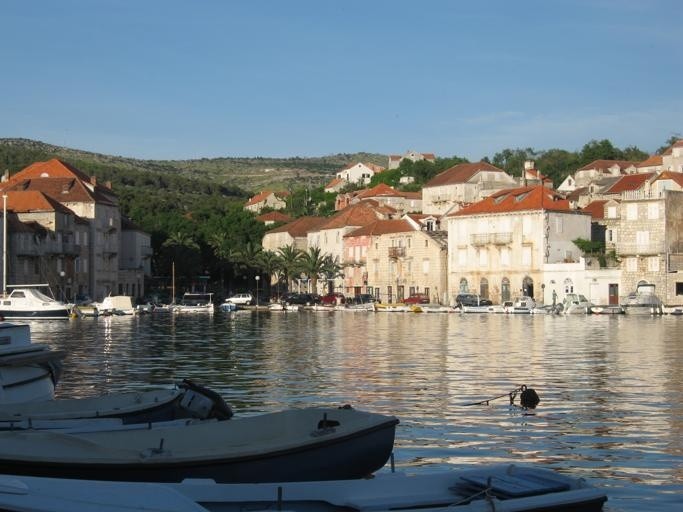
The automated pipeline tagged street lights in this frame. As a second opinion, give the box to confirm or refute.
[254,276,261,306]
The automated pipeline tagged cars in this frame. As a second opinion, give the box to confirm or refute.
[278,291,375,307]
[455,293,491,307]
[398,292,429,305]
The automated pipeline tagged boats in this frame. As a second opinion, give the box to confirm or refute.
[0,405,401,483]
[0,465,608,512]
[0,378,233,431]
[172,293,215,314]
[500,291,682,316]
[0,323,68,405]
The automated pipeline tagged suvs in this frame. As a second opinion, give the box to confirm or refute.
[224,293,254,306]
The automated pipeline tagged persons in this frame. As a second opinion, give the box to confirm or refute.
[551,289,559,308]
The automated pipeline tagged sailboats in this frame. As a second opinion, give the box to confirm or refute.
[0,188,70,321]
